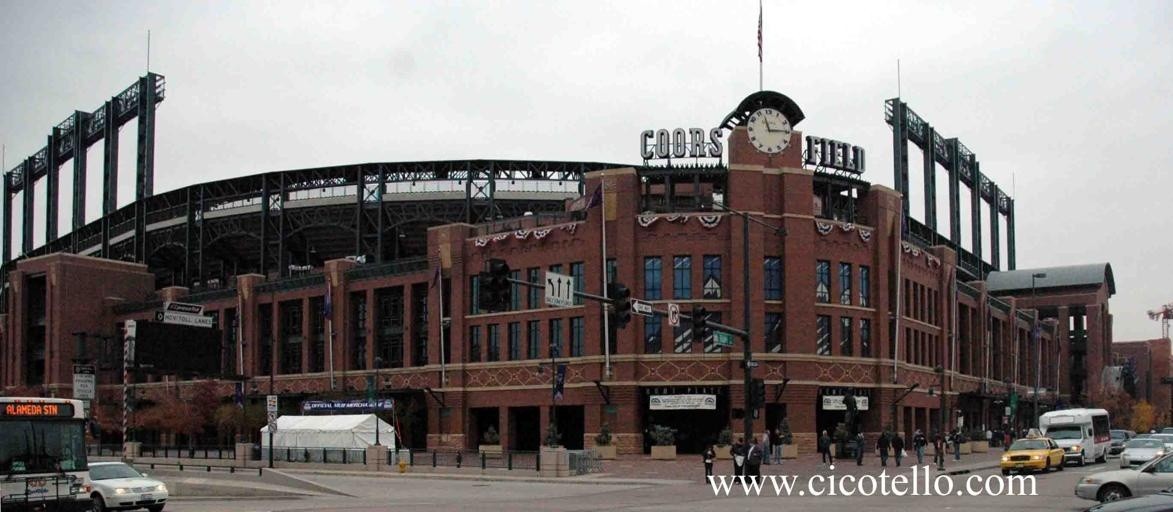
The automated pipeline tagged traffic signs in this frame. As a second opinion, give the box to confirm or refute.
[712,330,735,347]
[629,296,652,319]
[737,360,759,369]
[154,309,213,329]
[71,365,96,376]
[164,302,204,315]
[71,373,95,399]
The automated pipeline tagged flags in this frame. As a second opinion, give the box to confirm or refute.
[428,263,439,288]
[323,284,331,322]
[234,300,238,327]
[900,207,909,240]
[565,184,601,213]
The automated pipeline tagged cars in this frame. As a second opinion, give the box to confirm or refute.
[84,459,170,512]
[996,425,1172,503]
[1080,487,1172,512]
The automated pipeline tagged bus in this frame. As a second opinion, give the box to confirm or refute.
[0,395,93,512]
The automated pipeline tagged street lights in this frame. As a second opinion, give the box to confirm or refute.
[533,343,562,449]
[926,327,953,449]
[371,355,393,447]
[122,333,139,442]
[1029,272,1048,429]
[991,375,1015,430]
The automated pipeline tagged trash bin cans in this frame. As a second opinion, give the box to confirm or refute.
[399,449,410,465]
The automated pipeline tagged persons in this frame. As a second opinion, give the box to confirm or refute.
[842,387,857,428]
[747,437,761,483]
[730,438,744,484]
[818,430,832,465]
[701,444,714,483]
[986,426,1028,452]
[772,429,785,465]
[874,428,960,469]
[856,431,865,466]
[761,428,771,464]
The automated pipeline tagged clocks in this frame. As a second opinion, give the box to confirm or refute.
[746,107,793,158]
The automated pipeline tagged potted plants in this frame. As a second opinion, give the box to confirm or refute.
[713,426,733,459]
[479,426,503,458]
[772,417,798,459]
[650,424,677,460]
[591,422,616,460]
[924,426,989,456]
[828,423,851,457]
[539,423,564,456]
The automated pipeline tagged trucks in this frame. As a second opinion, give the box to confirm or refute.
[1036,408,1112,467]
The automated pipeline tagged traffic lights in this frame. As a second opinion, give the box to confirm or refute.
[750,407,759,419]
[610,282,631,330]
[730,408,743,420]
[486,254,513,313]
[690,302,709,344]
[476,270,501,311]
[749,378,765,409]
